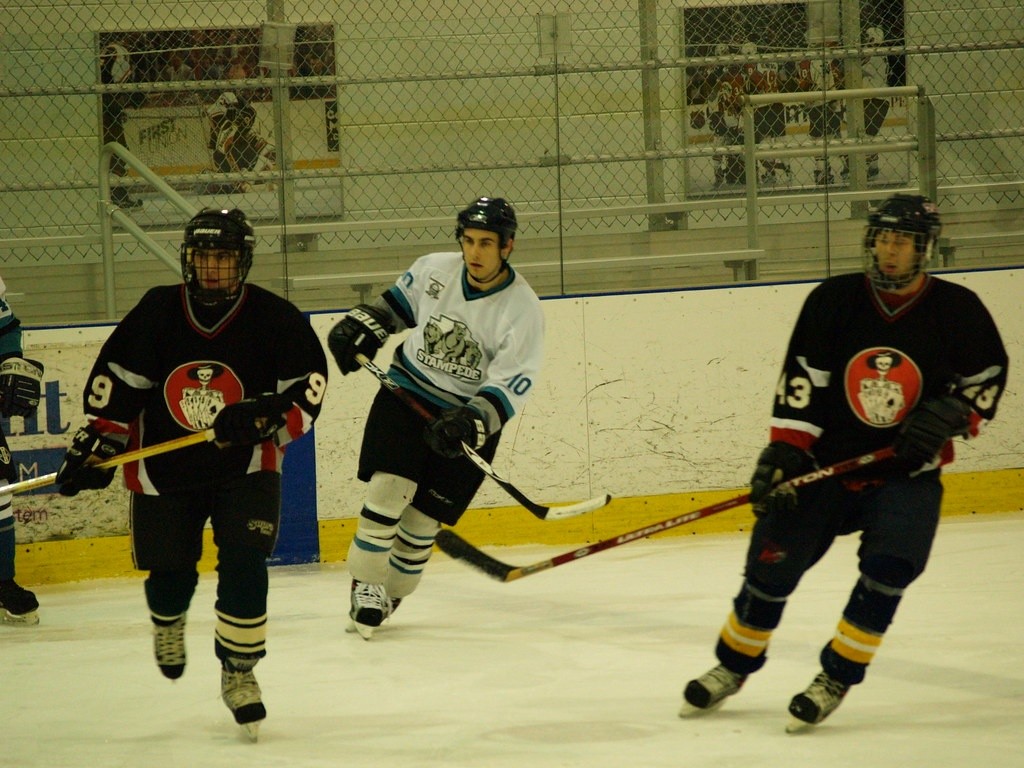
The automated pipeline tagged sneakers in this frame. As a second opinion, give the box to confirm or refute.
[347,577,404,642]
[681,664,745,718]
[151,624,188,683]
[0,582,42,629]
[788,672,851,734]
[220,667,267,741]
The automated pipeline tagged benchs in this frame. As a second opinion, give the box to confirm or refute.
[939,229,1024,267]
[270,249,766,306]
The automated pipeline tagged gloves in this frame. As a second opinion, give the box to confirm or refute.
[56,429,128,496]
[328,304,395,374]
[894,394,967,466]
[423,404,487,456]
[750,441,809,514]
[1,357,46,419]
[213,399,285,446]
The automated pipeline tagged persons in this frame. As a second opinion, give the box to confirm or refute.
[98,35,145,210]
[327,196,545,628]
[686,30,892,187]
[198,88,276,195]
[56,207,328,725]
[0,272,43,616]
[144,32,334,104]
[684,193,1011,726]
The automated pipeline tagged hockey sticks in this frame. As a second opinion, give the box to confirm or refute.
[431,447,893,584]
[0,428,218,497]
[358,351,613,522]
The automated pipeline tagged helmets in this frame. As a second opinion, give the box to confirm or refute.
[456,197,518,249]
[181,206,255,299]
[859,194,941,286]
